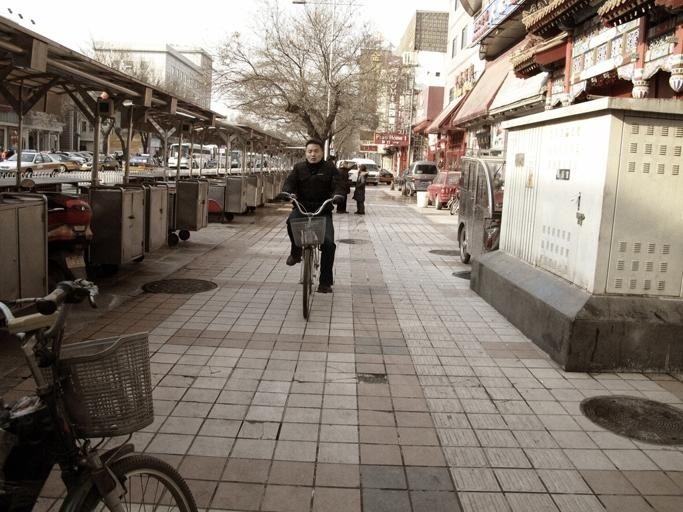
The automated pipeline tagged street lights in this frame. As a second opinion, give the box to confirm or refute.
[293,0,335,162]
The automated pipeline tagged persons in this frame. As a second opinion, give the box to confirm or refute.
[335,159,356,213]
[280,140,346,293]
[351,163,367,214]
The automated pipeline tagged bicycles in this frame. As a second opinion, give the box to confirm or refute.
[280,192,344,318]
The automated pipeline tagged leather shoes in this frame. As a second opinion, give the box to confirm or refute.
[318,284,331,293]
[286,255,297,266]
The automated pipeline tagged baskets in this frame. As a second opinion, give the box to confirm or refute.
[42,331,153,438]
[289,216,326,247]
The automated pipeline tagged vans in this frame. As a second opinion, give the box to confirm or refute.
[336,158,380,186]
[397,161,437,196]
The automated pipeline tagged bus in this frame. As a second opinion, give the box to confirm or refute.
[168,143,281,169]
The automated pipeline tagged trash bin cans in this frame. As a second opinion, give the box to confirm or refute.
[417,191,430,208]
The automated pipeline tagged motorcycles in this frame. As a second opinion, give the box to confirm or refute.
[48,195,96,302]
[0,279,198,512]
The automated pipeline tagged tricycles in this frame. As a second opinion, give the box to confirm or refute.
[458,156,506,263]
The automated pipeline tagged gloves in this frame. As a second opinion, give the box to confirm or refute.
[332,196,343,204]
[279,193,290,201]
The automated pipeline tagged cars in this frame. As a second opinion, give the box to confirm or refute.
[427,171,462,210]
[379,169,393,185]
[0,151,154,178]
